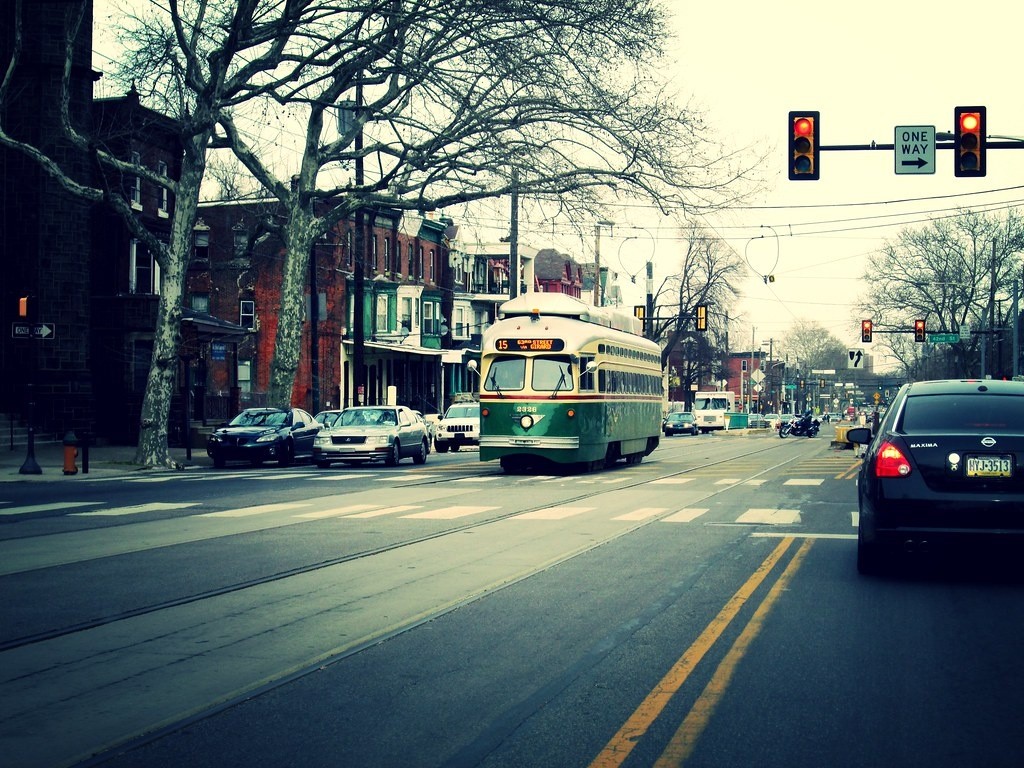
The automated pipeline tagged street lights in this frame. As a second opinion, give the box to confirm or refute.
[915,281,1020,382]
[936,130,1024,144]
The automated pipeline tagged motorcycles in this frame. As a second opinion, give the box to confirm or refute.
[779,413,820,439]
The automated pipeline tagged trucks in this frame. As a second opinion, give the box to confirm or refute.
[694,390,736,434]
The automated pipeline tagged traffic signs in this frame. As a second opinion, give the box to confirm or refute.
[11,321,56,341]
[893,126,936,174]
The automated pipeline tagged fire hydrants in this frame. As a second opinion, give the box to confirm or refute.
[63,430,81,476]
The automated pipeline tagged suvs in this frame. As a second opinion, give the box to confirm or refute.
[747,414,763,428]
[434,401,480,454]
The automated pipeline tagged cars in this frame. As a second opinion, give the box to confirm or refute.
[314,409,342,429]
[845,379,1024,579]
[207,406,325,469]
[413,411,432,454]
[764,414,779,427]
[663,413,671,432]
[313,404,428,467]
[666,412,699,437]
[780,414,792,422]
[811,407,875,423]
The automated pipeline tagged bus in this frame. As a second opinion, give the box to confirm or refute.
[467,290,664,478]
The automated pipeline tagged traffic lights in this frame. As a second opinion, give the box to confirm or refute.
[952,105,988,179]
[915,319,926,343]
[787,111,820,180]
[862,320,872,342]
[742,360,747,372]
